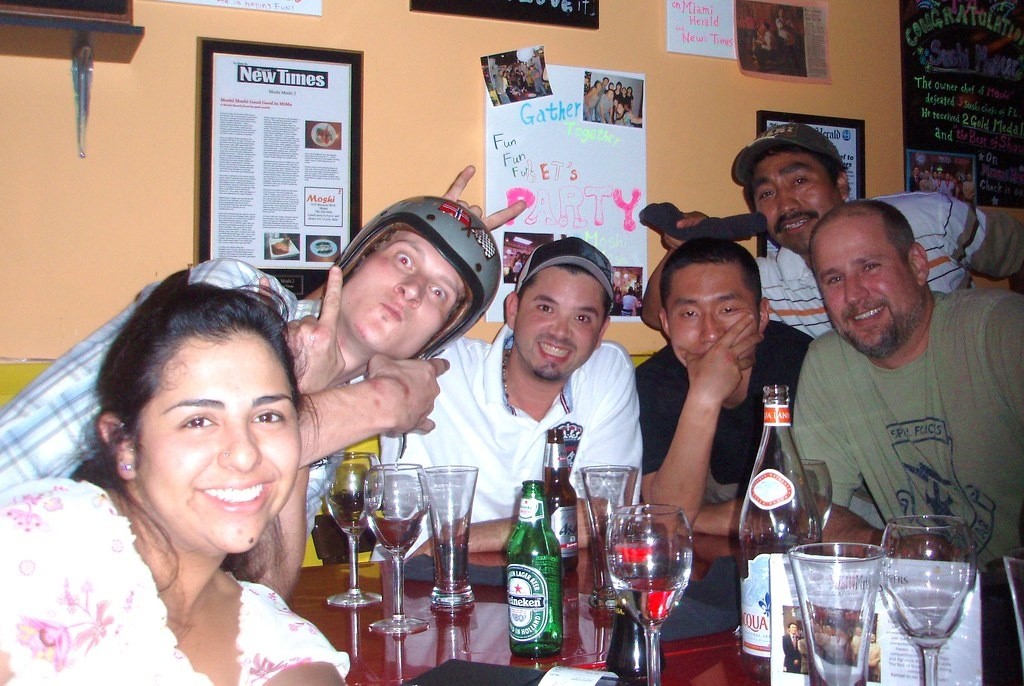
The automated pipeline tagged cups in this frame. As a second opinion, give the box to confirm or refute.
[417,466,479,605]
[788,542,885,686]
[770,459,832,538]
[581,465,639,609]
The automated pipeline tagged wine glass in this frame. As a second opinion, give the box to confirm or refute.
[881,515,976,686]
[604,504,694,686]
[364,464,429,634]
[325,453,382,608]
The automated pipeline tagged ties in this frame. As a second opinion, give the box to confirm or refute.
[792,636,794,639]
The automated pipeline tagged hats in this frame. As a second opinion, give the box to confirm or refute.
[514,235,615,314]
[730,126,844,196]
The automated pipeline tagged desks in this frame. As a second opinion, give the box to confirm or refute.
[289,553,769,686]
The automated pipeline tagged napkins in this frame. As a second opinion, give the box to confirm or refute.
[402,659,545,686]
[404,552,565,586]
[655,556,740,640]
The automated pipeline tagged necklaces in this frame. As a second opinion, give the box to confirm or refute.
[502,349,511,405]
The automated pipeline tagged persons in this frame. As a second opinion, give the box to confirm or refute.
[583,76,643,128]
[636,238,813,539]
[791,199,1024,580]
[0,283,351,686]
[380,165,643,558]
[910,166,974,202]
[640,124,1024,341]
[0,196,501,605]
[783,623,881,683]
[746,8,799,75]
[482,58,551,105]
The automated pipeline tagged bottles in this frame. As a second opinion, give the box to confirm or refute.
[544,428,578,576]
[738,386,821,682]
[606,591,665,681]
[506,480,563,658]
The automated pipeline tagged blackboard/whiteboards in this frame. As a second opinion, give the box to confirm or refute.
[899,0,1024,209]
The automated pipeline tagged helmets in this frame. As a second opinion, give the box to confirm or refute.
[331,195,502,360]
